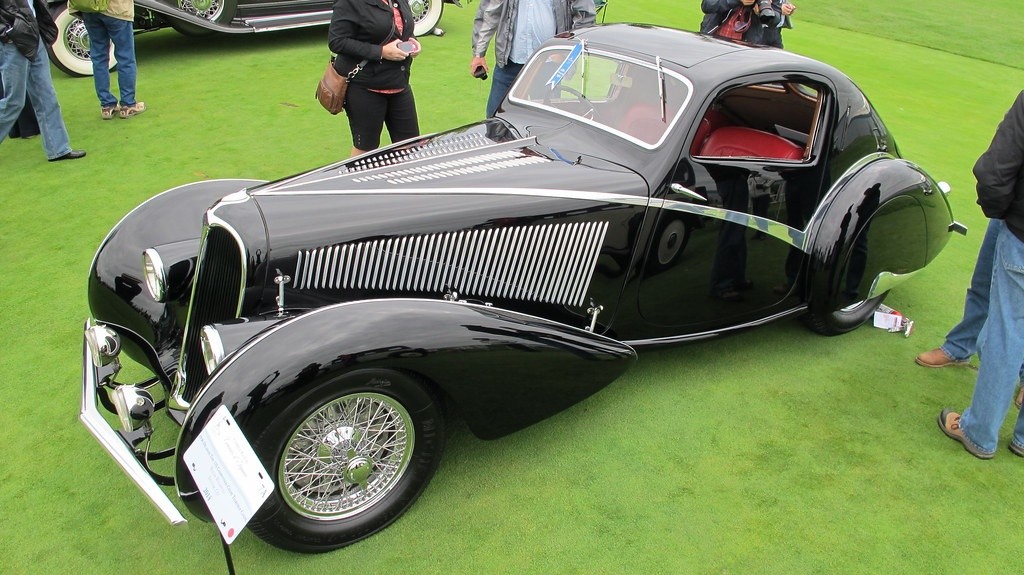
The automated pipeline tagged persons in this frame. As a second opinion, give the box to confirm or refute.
[704,166,880,309]
[916,218,1024,410]
[937,89,1024,459]
[700,0,795,49]
[0,0,86,162]
[470,0,596,119]
[67,0,146,120]
[327,0,421,158]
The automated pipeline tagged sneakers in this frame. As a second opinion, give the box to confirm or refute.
[116,101,145,118]
[1009,439,1024,457]
[101,105,121,121]
[938,408,995,461]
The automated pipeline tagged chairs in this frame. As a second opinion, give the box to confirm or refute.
[699,126,805,160]
[616,106,710,156]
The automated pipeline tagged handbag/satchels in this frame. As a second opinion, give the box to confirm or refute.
[312,59,353,115]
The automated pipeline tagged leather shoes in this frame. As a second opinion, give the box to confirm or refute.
[49,150,86,160]
[915,348,971,367]
[1016,385,1023,411]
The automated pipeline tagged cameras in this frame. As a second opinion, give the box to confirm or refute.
[755,0,776,25]
[397,41,418,54]
[474,66,488,80]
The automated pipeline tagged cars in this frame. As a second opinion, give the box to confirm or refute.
[77,24,969,556]
[43,1,448,80]
